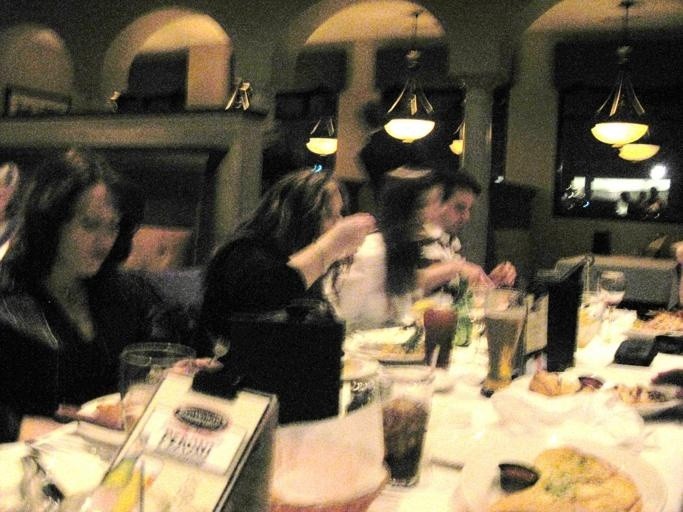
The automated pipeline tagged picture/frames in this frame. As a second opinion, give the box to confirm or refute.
[2,83,72,120]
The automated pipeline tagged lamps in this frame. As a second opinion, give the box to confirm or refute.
[382,11,436,146]
[590,0,659,162]
[305,115,337,157]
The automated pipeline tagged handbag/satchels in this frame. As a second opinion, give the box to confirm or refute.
[217,298,345,427]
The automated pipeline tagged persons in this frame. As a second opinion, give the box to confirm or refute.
[195,169,377,357]
[614,187,664,218]
[673,240,683,308]
[414,167,519,304]
[336,169,442,330]
[0,145,225,446]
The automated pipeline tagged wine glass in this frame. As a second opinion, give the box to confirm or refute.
[597,269,626,332]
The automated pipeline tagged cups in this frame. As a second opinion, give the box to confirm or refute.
[379,377,433,489]
[114,454,164,512]
[481,288,529,390]
[424,306,458,370]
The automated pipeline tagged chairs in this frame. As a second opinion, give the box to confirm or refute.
[117,265,203,347]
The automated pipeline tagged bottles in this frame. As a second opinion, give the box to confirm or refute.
[101,430,149,490]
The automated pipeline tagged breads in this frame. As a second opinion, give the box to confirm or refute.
[480,448,642,512]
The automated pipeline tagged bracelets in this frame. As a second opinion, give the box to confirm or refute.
[312,236,328,277]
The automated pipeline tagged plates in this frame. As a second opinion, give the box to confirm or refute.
[77,393,127,446]
[461,436,667,511]
[509,372,682,418]
[340,326,425,381]
